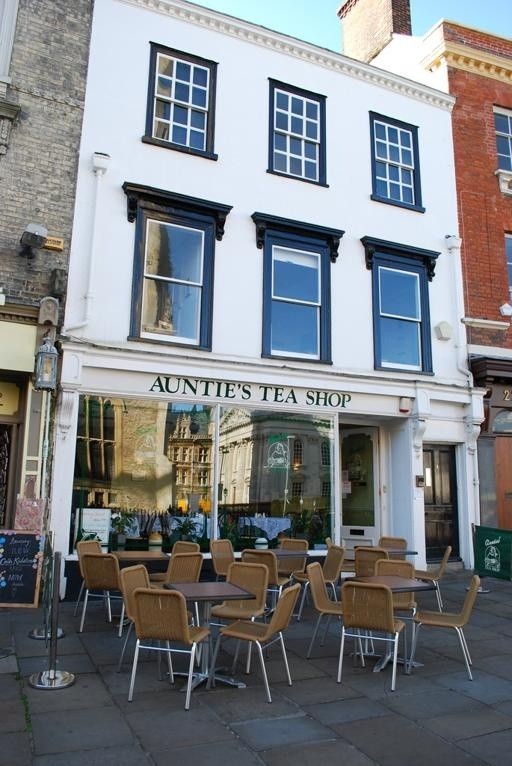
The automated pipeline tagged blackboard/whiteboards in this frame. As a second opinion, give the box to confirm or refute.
[0,529,47,609]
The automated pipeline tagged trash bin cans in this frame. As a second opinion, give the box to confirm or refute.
[473,525,512,582]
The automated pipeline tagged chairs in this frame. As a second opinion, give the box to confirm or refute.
[72,541,112,617]
[405,575,480,680]
[241,550,293,615]
[126,588,215,711]
[413,545,452,614]
[336,580,406,692]
[378,536,408,561]
[212,562,270,673]
[152,552,204,588]
[210,539,236,581]
[353,547,389,578]
[148,541,200,580]
[373,559,418,652]
[305,562,361,668]
[115,564,167,681]
[206,582,303,703]
[276,538,310,586]
[293,545,345,621]
[77,552,130,638]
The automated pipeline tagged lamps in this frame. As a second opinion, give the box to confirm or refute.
[32,326,58,392]
[19,223,49,261]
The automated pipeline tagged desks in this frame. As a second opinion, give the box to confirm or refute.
[161,583,256,695]
[112,550,169,563]
[344,575,438,672]
[235,547,311,562]
[342,549,418,563]
